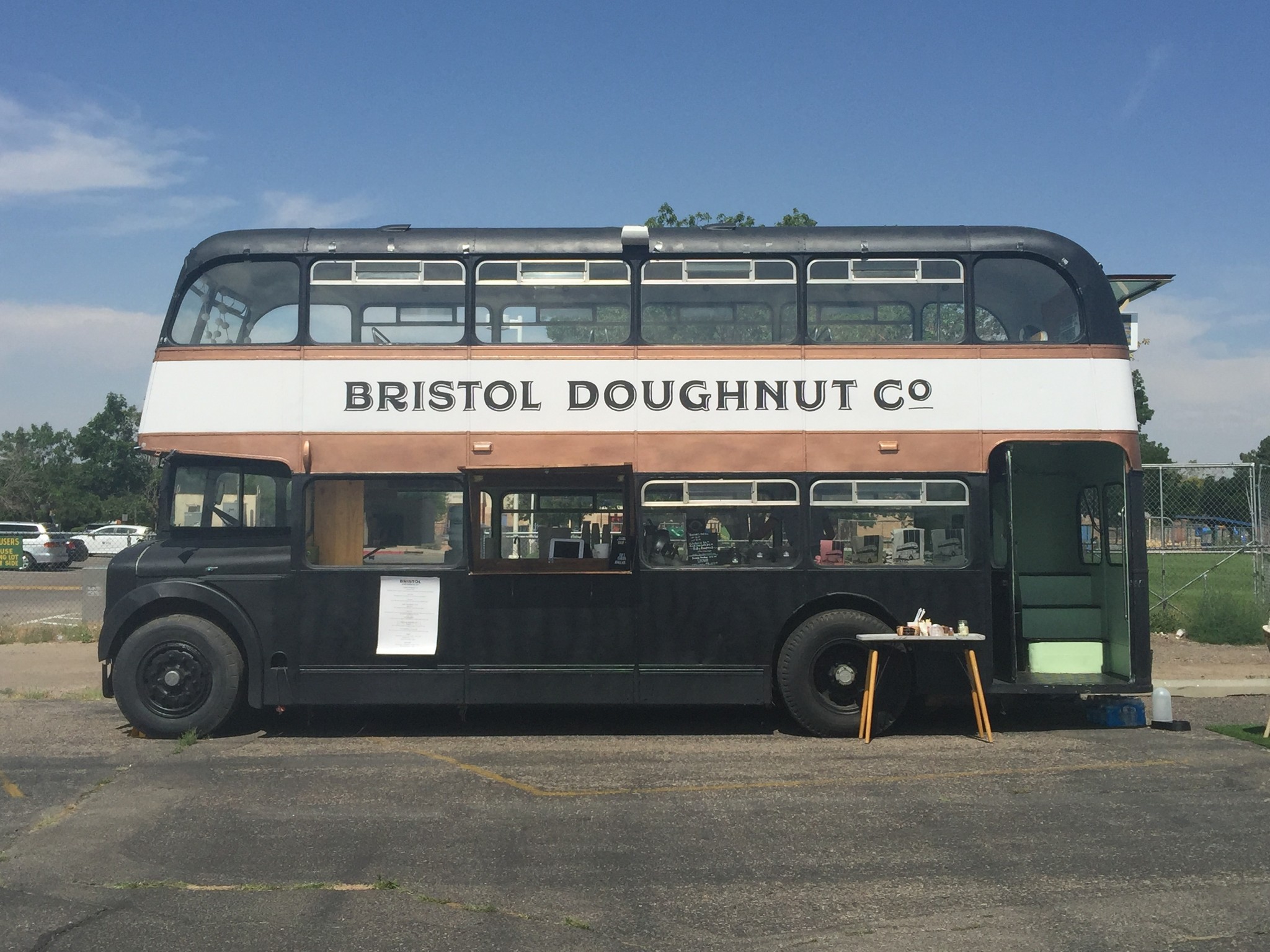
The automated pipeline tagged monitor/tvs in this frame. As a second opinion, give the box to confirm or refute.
[548,539,584,559]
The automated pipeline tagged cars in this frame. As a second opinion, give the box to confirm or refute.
[65,525,155,558]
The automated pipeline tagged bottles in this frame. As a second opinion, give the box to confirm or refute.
[896,620,969,636]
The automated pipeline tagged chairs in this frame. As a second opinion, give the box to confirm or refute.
[369,327,832,346]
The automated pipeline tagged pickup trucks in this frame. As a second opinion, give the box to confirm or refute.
[65,537,89,566]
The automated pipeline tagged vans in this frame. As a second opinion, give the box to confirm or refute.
[0,522,69,571]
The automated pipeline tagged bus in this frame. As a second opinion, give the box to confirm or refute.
[98,224,1177,739]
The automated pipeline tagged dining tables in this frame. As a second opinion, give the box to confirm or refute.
[855,633,994,742]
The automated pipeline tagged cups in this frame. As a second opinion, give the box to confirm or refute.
[594,543,609,559]
